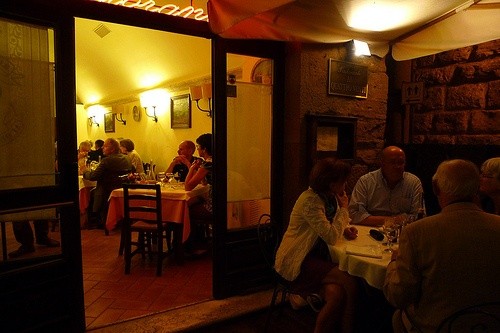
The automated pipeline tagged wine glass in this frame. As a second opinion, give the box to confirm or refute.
[384,226,399,254]
[158,173,175,189]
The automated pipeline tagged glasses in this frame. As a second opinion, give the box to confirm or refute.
[382,159,405,164]
[481,171,496,179]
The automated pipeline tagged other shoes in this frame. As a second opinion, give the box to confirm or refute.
[8,245,34,257]
[187,251,207,260]
[36,238,58,247]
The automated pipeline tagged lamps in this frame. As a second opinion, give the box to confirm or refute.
[189,83,213,119]
[139,89,161,123]
[86,104,102,127]
[111,103,127,126]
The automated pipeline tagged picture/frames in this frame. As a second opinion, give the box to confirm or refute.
[327,57,369,99]
[169,93,192,129]
[103,112,116,133]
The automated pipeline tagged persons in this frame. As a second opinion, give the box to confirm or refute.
[166,142,204,182]
[479,157,500,216]
[349,146,427,228]
[9,218,60,256]
[382,160,500,333]
[185,133,212,244]
[273,157,356,333]
[78,138,146,217]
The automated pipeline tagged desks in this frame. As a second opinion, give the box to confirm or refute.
[105,181,212,268]
[326,224,400,291]
[78,174,98,229]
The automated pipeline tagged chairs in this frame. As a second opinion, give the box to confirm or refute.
[253,212,321,333]
[122,182,184,277]
[435,299,500,333]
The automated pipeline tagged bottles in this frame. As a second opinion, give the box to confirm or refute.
[416,209,424,221]
[86,157,91,171]
